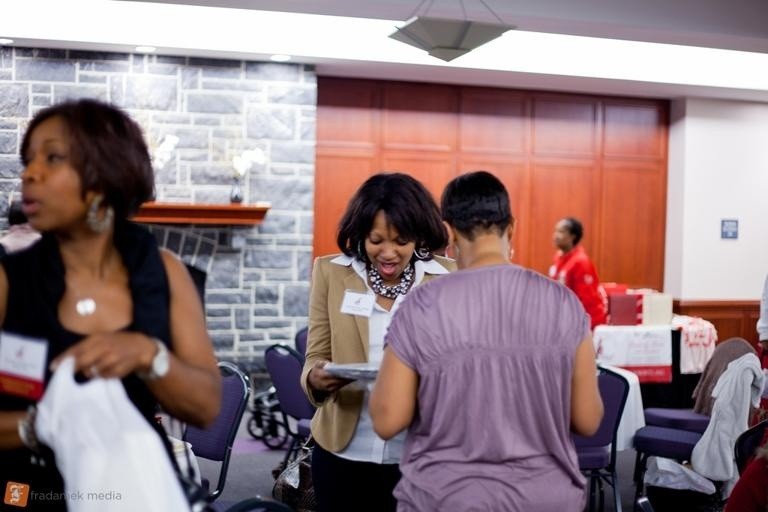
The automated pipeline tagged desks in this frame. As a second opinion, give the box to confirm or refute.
[588,313,718,377]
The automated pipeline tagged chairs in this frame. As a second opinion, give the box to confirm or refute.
[635,423,743,512]
[181,358,251,504]
[645,337,756,431]
[295,329,309,353]
[568,365,629,510]
[262,345,316,481]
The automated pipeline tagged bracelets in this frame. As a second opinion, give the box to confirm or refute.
[18,407,39,448]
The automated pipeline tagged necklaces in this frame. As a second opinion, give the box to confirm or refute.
[62,250,113,316]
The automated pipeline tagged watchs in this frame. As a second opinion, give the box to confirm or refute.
[135,337,170,382]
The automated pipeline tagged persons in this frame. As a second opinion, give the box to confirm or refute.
[549,217,605,329]
[0,97,223,511]
[299,173,459,512]
[369,171,605,512]
[755,273,767,357]
